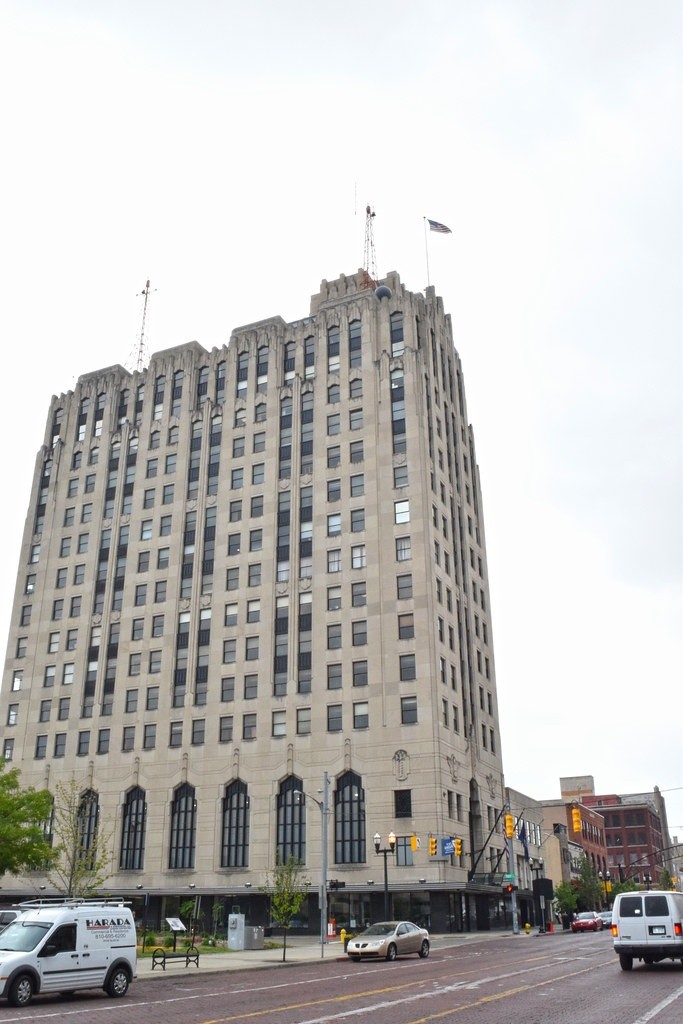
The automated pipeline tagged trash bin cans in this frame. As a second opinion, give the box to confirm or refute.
[561,914,570,930]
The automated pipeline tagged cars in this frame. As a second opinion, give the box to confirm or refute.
[598,911,612,929]
[571,911,603,933]
[346,921,430,962]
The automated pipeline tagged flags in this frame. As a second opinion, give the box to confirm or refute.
[428,219,452,233]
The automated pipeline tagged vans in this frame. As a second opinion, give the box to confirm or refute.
[611,890,683,970]
[0,897,137,1007]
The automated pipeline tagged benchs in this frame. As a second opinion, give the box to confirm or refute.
[151,946,199,970]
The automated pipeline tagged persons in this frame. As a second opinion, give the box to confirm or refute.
[566,910,579,926]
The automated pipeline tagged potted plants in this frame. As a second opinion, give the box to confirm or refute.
[552,879,579,929]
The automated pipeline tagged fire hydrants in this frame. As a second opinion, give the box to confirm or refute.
[339,928,352,944]
[525,923,530,934]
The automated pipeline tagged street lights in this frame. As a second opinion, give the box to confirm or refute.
[598,870,611,910]
[528,856,547,932]
[642,875,652,889]
[372,829,396,921]
[292,790,322,807]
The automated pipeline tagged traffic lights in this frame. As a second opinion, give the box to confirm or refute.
[429,838,436,856]
[507,885,514,892]
[454,839,461,856]
